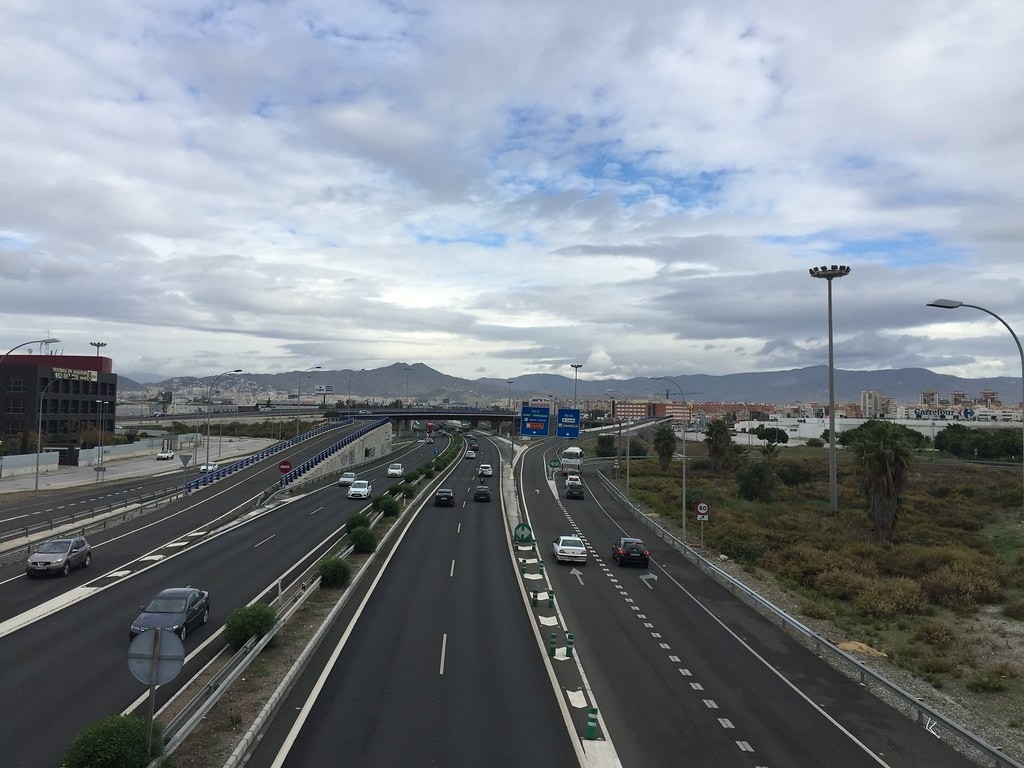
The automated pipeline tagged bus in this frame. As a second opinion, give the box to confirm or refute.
[562,447,584,473]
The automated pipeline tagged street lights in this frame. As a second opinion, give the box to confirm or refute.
[810,263,851,517]
[90,341,109,482]
[571,364,582,409]
[297,366,411,438]
[35,376,75,493]
[507,381,513,409]
[207,369,243,479]
[605,376,686,541]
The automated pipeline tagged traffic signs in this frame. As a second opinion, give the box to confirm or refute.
[520,406,550,437]
[556,409,580,438]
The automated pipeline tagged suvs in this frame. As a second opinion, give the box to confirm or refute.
[435,464,493,507]
[359,410,372,415]
[564,470,584,499]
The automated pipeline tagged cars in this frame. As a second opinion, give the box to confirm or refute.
[26,535,210,642]
[157,450,218,473]
[553,536,650,566]
[259,408,270,411]
[339,464,404,499]
[425,419,479,459]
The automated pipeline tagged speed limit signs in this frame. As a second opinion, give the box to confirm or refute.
[696,501,709,515]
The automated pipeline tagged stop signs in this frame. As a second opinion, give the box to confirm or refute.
[279,461,291,473]
